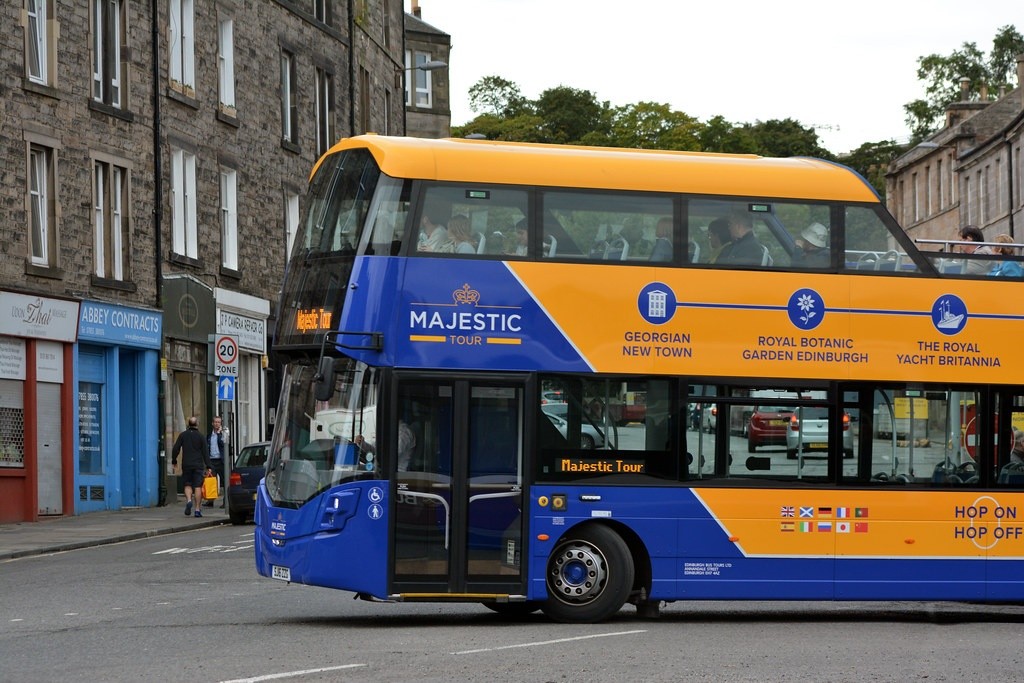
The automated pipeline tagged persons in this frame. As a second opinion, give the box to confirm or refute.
[417,201,477,253]
[706,209,768,266]
[202,416,225,508]
[648,217,673,262]
[503,218,528,256]
[987,234,1024,277]
[172,417,212,517]
[958,225,995,274]
[791,222,831,268]
[353,399,416,472]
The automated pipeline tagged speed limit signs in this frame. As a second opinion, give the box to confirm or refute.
[216,337,237,364]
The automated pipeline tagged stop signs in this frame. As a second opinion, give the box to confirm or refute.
[965,411,1016,467]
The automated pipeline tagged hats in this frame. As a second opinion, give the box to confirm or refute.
[800,222,828,247]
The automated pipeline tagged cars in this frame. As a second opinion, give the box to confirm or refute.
[746,406,804,454]
[541,391,756,437]
[782,403,855,460]
[227,441,277,525]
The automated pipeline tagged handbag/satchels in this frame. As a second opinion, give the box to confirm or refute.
[201,471,218,499]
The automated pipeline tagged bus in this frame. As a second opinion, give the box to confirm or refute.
[250,133,1024,624]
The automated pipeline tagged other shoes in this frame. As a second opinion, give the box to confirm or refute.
[184,501,193,516]
[219,504,226,508]
[203,501,213,507]
[195,508,202,518]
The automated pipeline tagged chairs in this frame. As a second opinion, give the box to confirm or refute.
[872,459,1023,490]
[250,456,268,465]
[416,223,1023,278]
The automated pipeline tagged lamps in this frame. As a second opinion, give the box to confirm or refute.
[918,142,955,149]
[390,58,448,74]
